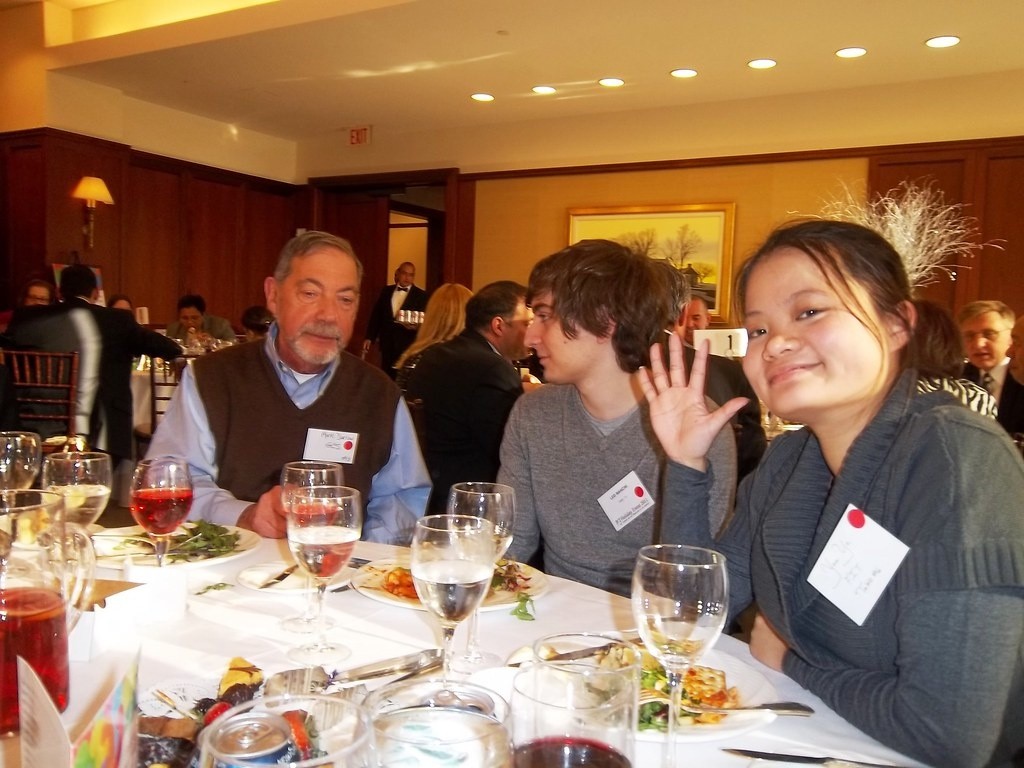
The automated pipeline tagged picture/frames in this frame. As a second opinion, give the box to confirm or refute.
[562,200,738,323]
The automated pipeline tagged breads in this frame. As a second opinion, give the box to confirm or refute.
[217,655,263,700]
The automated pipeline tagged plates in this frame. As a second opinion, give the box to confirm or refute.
[236,559,356,594]
[91,524,263,571]
[605,633,777,742]
[351,553,550,612]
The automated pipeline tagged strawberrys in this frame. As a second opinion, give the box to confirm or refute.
[203,701,234,726]
[279,709,318,759]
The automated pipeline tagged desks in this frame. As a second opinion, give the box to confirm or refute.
[131,340,237,435]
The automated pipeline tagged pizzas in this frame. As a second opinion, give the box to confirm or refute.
[387,566,418,599]
[682,666,728,708]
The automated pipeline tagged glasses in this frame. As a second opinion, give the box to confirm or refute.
[28,294,49,304]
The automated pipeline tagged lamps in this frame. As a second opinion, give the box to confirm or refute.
[73,176,114,248]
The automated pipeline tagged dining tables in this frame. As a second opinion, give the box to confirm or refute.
[0,527,923,768]
[134,353,196,459]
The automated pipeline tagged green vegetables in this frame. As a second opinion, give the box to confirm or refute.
[583,660,704,735]
[489,557,538,621]
[108,518,241,564]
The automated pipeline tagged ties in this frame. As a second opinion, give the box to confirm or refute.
[982,372,990,388]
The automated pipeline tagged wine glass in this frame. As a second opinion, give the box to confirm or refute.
[410,515,495,707]
[447,482,517,674]
[632,544,729,768]
[287,485,363,665]
[279,462,345,634]
[0,431,41,507]
[129,456,194,568]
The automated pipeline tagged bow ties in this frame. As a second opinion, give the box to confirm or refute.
[397,287,409,292]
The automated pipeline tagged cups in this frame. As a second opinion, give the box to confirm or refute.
[504,663,640,768]
[532,632,644,750]
[196,694,378,768]
[0,490,97,740]
[168,338,234,363]
[350,677,515,768]
[42,451,112,529]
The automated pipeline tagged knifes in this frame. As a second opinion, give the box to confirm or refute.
[721,748,898,768]
[257,564,298,588]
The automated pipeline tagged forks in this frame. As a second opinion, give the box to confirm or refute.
[592,689,815,722]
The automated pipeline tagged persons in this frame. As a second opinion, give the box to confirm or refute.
[911,299,997,419]
[362,262,429,377]
[684,293,710,346]
[0,279,56,334]
[636,177,1024,768]
[391,281,476,388]
[166,294,235,345]
[653,259,767,477]
[954,301,1024,433]
[106,296,132,309]
[484,240,738,598]
[242,306,273,341]
[1006,315,1024,385]
[138,231,432,545]
[401,281,534,546]
[0,266,180,468]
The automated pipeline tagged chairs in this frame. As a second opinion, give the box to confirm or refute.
[0,349,81,471]
